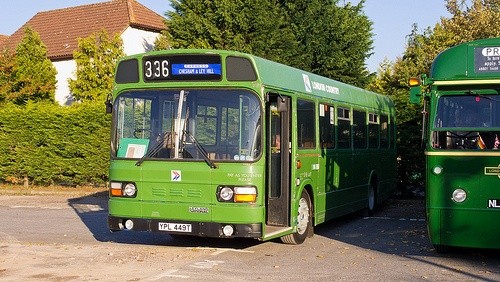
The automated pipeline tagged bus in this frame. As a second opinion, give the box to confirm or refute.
[408,38,500,253]
[107,48,397,247]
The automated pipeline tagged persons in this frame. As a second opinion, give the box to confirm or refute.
[445,105,499,150]
